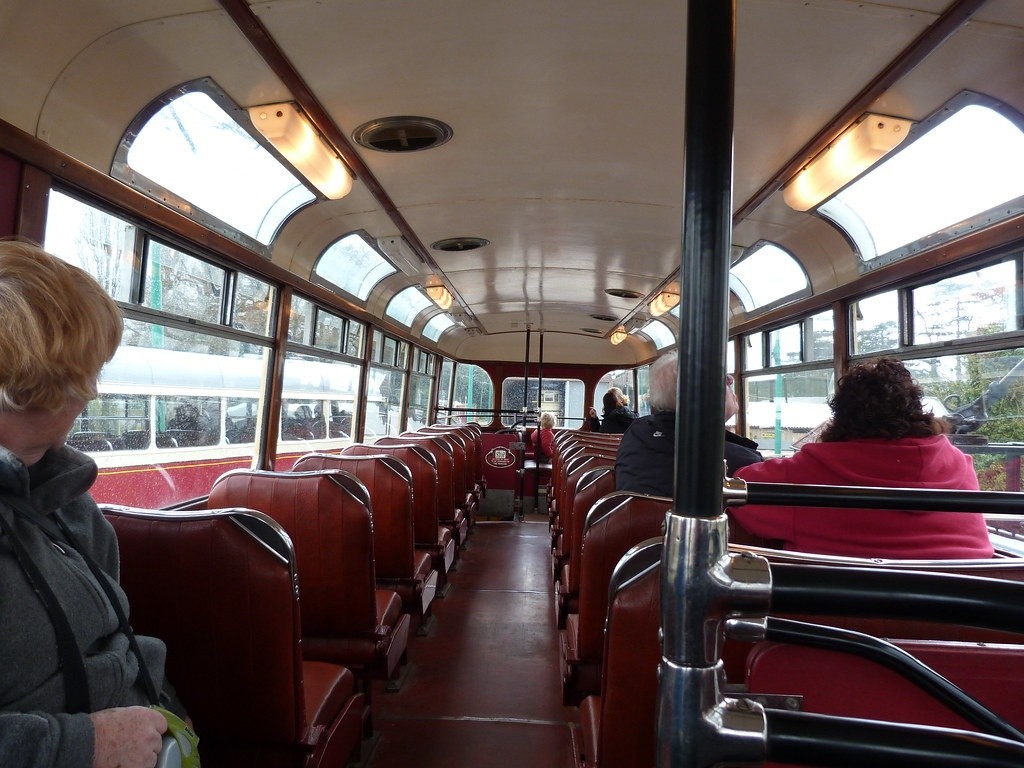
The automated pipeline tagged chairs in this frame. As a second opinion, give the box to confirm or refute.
[86,421,525,768]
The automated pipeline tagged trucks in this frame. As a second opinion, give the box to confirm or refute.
[502,377,613,428]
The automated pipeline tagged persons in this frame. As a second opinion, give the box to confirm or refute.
[614,353,764,498]
[531,413,556,464]
[0,241,193,768]
[168,406,209,447]
[728,358,995,560]
[283,403,348,440]
[589,387,640,434]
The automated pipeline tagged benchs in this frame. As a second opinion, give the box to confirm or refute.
[548,424,1024,768]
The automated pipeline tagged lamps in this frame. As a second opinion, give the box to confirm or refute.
[424,284,455,309]
[610,331,627,345]
[240,98,356,199]
[647,292,680,318]
[779,111,919,213]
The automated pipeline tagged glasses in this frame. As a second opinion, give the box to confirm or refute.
[726,374,734,385]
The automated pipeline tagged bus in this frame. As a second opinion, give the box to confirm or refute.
[67,344,391,508]
